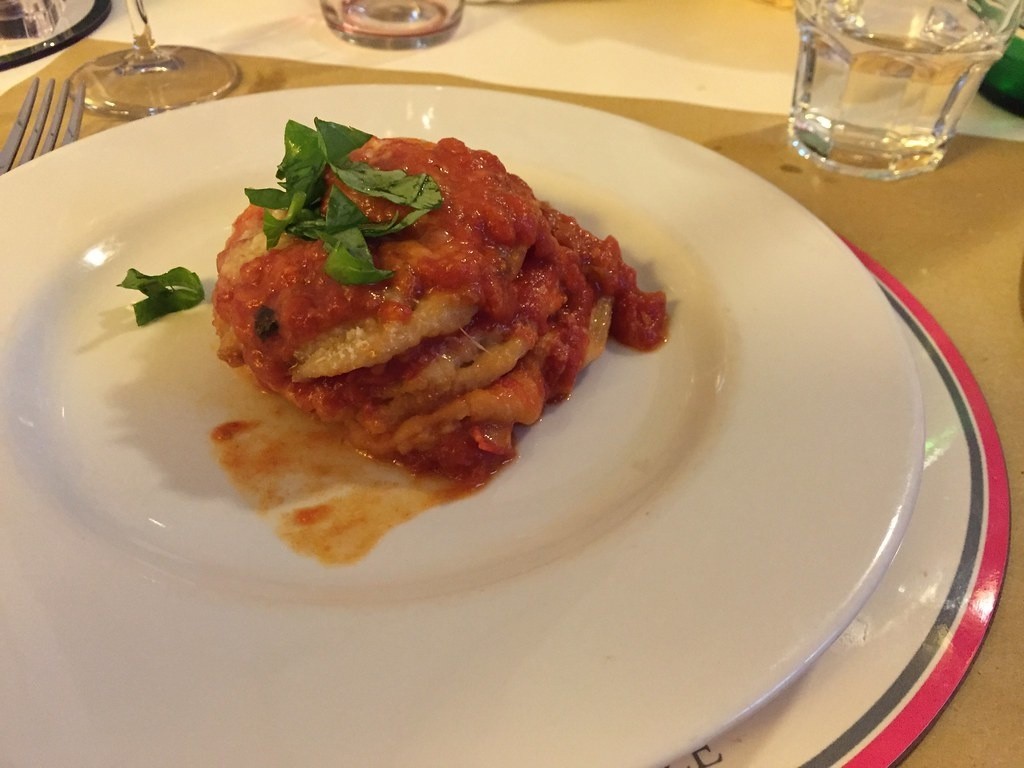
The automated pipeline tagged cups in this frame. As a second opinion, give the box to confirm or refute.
[786,0,1024,180]
[321,1,465,51]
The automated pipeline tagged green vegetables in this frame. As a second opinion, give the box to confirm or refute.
[118,116,444,326]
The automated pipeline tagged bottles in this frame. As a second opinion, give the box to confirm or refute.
[967,0,1024,117]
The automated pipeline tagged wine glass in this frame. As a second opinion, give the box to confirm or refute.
[64,0,241,120]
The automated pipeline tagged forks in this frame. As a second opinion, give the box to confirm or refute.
[0,77,85,178]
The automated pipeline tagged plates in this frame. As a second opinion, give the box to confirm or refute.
[0,83,921,768]
